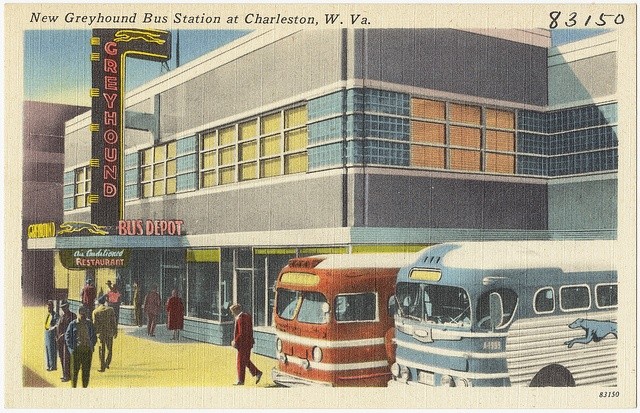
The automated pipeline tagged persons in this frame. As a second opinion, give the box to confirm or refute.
[91,295,116,372]
[45,300,59,372]
[166,289,184,340]
[59,299,77,383]
[132,282,142,327]
[105,283,120,324]
[65,305,97,389]
[143,285,160,337]
[229,304,263,386]
[106,279,113,293]
[79,279,95,319]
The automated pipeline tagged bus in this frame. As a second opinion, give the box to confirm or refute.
[387,240,617,388]
[271,252,416,387]
